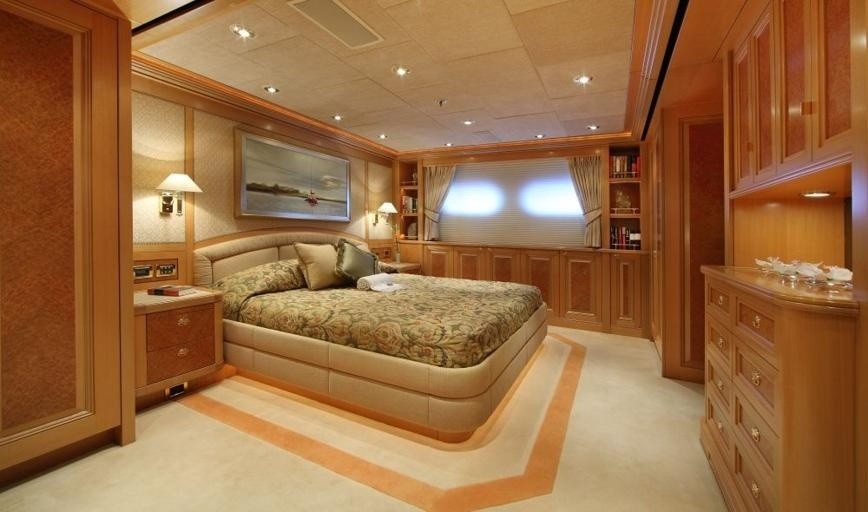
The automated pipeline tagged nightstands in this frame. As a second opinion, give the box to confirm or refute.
[382,261,422,275]
[134,283,226,395]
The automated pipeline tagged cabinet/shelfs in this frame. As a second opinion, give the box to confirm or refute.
[699,278,860,512]
[1,0,146,472]
[725,5,861,181]
[598,143,651,251]
[396,156,425,240]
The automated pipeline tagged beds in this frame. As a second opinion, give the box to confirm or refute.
[183,227,548,447]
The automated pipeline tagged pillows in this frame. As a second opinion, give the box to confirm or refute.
[289,236,380,291]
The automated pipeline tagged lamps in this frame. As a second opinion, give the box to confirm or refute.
[157,170,205,215]
[374,203,399,225]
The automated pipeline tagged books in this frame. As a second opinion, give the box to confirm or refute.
[610,224,641,250]
[400,195,418,214]
[147,284,195,297]
[609,154,641,178]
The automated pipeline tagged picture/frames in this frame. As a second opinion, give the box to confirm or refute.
[233,128,353,224]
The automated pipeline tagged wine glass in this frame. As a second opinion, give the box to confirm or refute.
[807,256,824,284]
